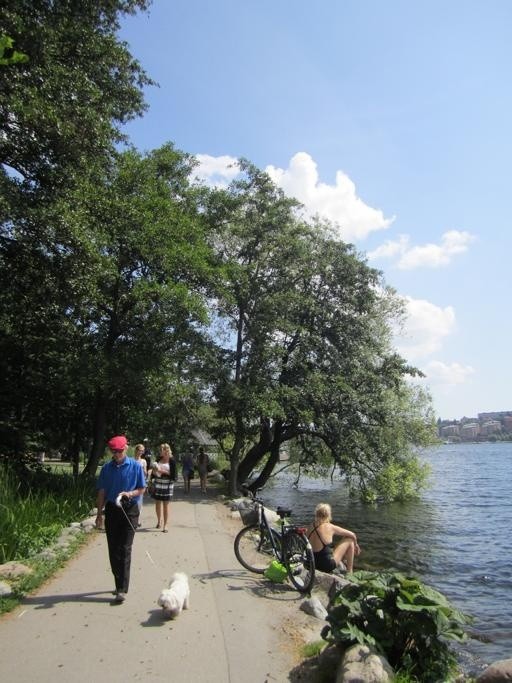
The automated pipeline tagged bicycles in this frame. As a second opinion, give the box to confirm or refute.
[226,483,316,594]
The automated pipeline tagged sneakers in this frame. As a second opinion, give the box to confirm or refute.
[112,589,126,601]
[138,522,169,533]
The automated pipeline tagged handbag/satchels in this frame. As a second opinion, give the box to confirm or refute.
[188,469,194,479]
[207,464,213,472]
[148,477,156,497]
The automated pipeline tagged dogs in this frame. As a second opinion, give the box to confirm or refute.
[158,571,190,619]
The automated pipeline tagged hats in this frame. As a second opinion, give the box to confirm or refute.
[107,435,127,450]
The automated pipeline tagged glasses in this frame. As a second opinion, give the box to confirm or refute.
[111,449,124,454]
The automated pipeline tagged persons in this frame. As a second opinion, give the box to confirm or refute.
[94,434,146,603]
[128,443,148,526]
[141,445,151,479]
[150,442,178,532]
[301,501,362,577]
[179,447,196,495]
[196,446,210,495]
